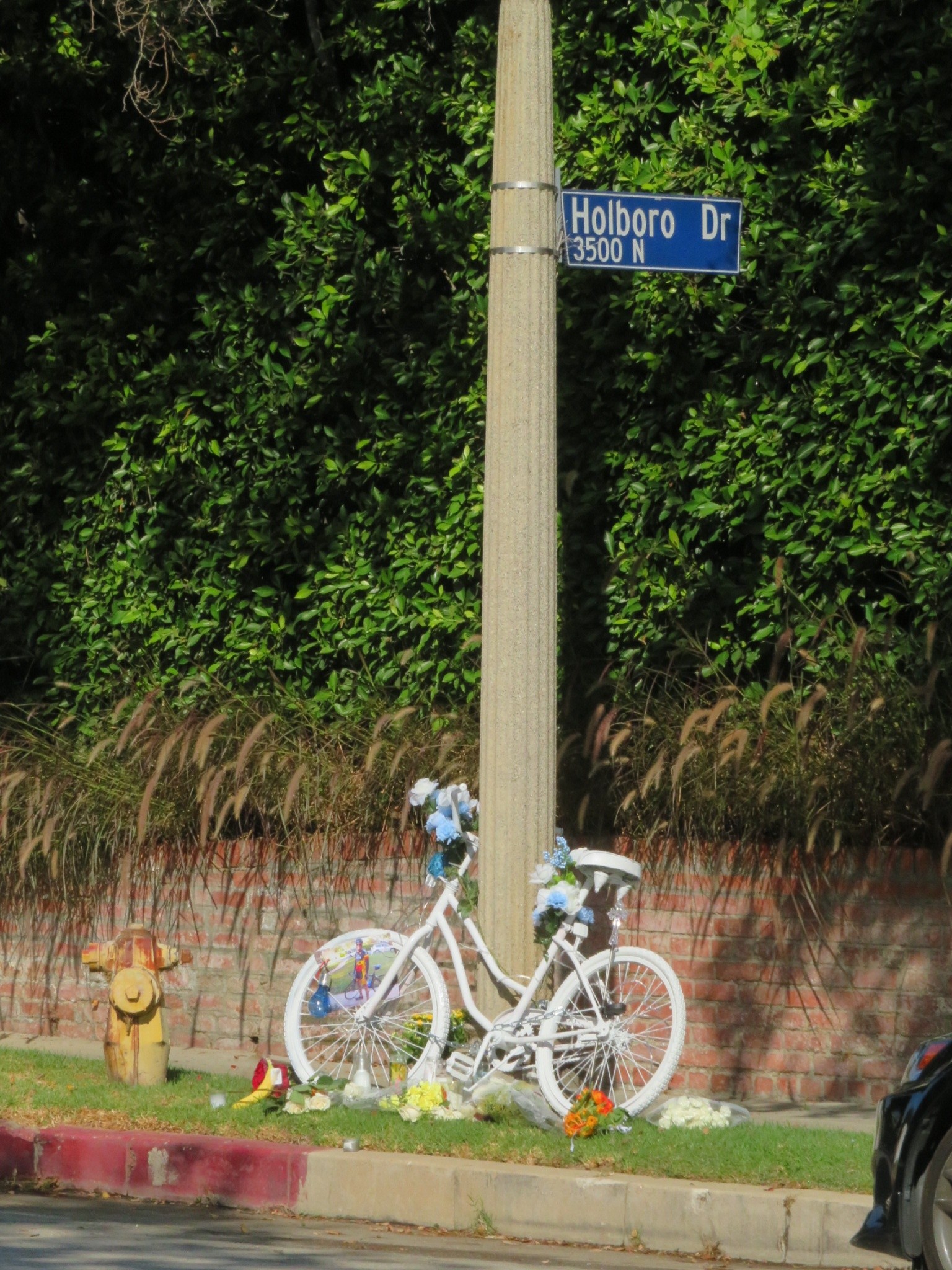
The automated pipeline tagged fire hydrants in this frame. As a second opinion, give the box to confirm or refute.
[81,920,192,1086]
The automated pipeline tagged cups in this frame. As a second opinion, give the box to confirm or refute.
[343,1138,360,1153]
[210,1093,226,1110]
[449,1080,465,1112]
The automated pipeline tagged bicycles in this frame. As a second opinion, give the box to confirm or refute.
[282,776,688,1123]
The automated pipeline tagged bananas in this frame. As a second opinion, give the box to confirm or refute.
[232,1058,275,1108]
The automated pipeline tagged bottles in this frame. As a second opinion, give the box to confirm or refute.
[389,1052,409,1107]
[424,1057,437,1084]
[352,1051,372,1090]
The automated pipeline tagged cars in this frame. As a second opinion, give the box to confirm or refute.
[848,1030,952,1270]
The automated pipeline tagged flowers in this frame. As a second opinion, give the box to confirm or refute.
[564,1088,634,1139]
[223,1077,363,1114]
[409,778,512,980]
[379,1081,463,1120]
[402,1010,469,1065]
[527,836,595,1007]
